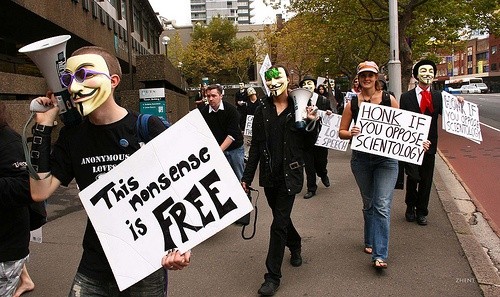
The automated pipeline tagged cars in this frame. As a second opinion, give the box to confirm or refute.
[460,85,481,94]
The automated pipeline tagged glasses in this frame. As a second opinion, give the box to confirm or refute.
[60,68,110,87]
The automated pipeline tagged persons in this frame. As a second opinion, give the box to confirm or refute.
[375,74,396,99]
[317,77,361,115]
[295,75,331,199]
[235,81,248,133]
[29,46,191,297]
[0,100,48,297]
[240,87,262,163]
[394,60,464,226]
[240,65,320,296]
[200,84,246,183]
[339,60,431,268]
[194,81,209,111]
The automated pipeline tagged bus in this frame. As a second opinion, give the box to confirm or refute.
[445,78,483,95]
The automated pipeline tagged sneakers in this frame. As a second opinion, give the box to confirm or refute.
[290,250,302,266]
[258,278,280,296]
[303,191,314,199]
[321,175,330,187]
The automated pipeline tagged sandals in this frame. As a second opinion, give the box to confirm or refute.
[364,247,372,254]
[374,259,387,269]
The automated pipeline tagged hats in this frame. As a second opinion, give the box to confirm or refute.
[357,61,379,75]
[299,75,317,88]
[413,59,437,81]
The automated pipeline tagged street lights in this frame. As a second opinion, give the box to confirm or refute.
[161,36,170,59]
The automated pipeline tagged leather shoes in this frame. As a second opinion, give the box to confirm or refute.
[405,206,415,221]
[417,215,427,225]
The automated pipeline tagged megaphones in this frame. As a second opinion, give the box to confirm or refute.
[288,88,318,128]
[18,35,73,115]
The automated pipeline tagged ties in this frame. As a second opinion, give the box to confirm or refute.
[421,91,434,114]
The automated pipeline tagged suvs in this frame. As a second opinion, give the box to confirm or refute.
[471,82,488,94]
